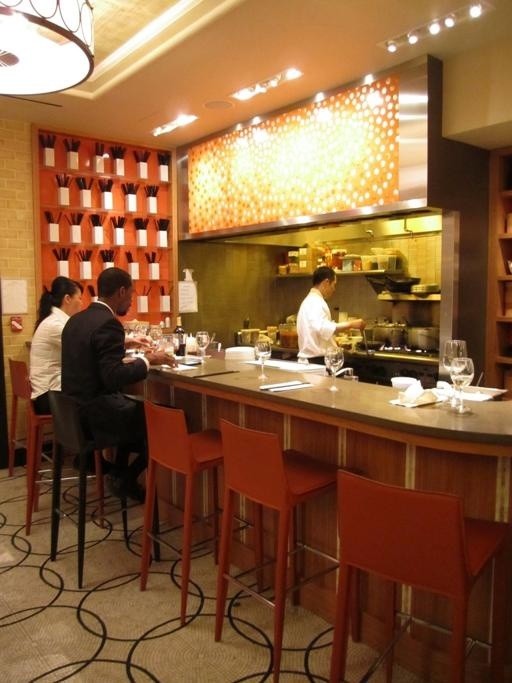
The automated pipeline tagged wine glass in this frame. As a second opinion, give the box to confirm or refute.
[254,339,272,381]
[324,345,345,392]
[195,331,210,369]
[442,339,475,417]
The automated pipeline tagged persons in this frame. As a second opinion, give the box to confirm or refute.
[26,274,118,475]
[60,266,181,506]
[295,265,367,382]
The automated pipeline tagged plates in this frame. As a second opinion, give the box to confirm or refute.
[410,283,440,294]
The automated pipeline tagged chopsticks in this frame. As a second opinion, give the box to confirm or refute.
[193,370,240,379]
[39,133,174,298]
[260,381,311,391]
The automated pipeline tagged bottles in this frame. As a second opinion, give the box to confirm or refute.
[173,316,186,356]
[237,326,278,346]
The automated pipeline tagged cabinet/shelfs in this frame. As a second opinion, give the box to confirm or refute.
[30,121,179,334]
[484,146,512,400]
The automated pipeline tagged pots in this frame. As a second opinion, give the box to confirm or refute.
[364,319,439,353]
[365,274,421,295]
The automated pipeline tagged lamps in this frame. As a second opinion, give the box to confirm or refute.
[1,0,95,96]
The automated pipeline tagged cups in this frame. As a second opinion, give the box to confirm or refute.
[279,323,298,349]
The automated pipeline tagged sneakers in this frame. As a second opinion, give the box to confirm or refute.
[107,474,144,500]
[74,454,114,474]
